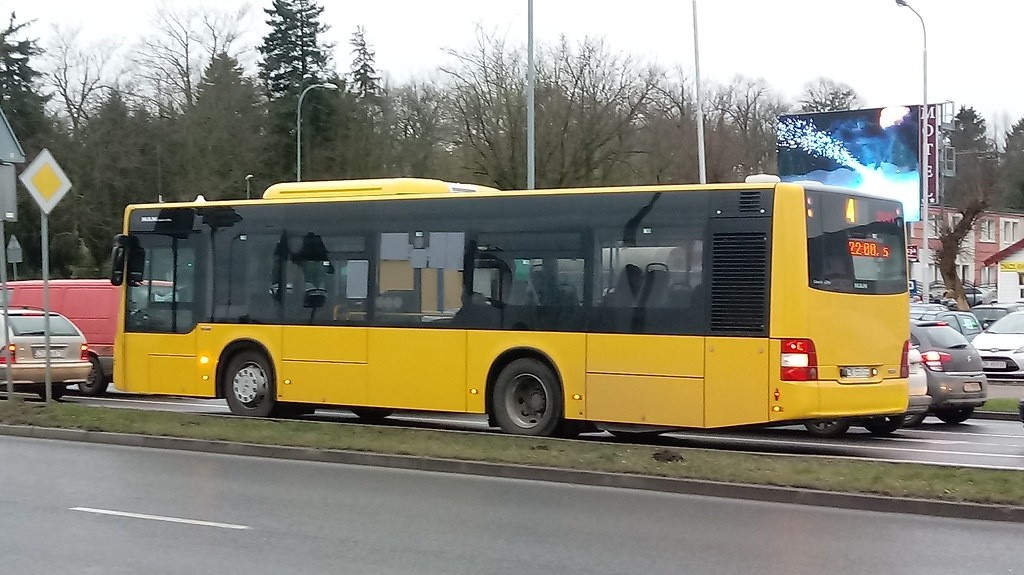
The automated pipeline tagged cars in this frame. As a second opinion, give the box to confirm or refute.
[2,307,92,401]
[911,277,1024,427]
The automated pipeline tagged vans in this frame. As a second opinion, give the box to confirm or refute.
[4,281,120,393]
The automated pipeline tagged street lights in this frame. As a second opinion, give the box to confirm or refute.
[241,173,254,198]
[293,79,341,181]
[899,0,932,300]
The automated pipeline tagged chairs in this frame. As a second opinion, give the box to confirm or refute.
[260,260,676,323]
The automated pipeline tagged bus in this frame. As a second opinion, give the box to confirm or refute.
[99,176,912,441]
